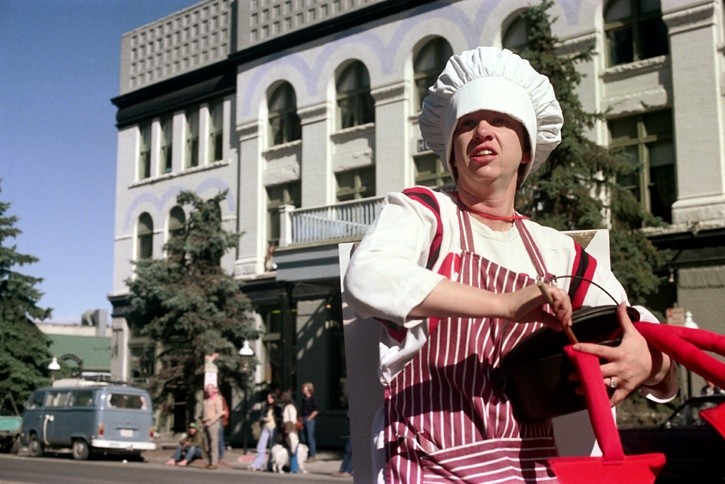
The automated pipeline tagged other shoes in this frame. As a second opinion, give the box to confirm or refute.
[166,460,175,465]
[206,465,217,469]
[331,471,352,478]
[177,462,187,466]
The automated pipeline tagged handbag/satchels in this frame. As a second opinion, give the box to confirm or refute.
[296,421,304,431]
[251,421,262,440]
[284,421,294,433]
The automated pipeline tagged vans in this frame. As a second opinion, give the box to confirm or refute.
[19,383,156,461]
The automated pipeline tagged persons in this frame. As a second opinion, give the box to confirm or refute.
[699,378,725,410]
[342,43,682,483]
[199,382,320,471]
[165,422,206,466]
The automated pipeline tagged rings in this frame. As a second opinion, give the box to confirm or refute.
[610,377,617,389]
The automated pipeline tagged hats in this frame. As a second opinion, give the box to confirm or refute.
[188,422,197,428]
[418,45,564,190]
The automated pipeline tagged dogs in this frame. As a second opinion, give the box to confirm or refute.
[270,443,309,474]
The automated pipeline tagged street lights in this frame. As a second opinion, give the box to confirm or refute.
[682,311,699,428]
[237,336,256,457]
[48,356,61,388]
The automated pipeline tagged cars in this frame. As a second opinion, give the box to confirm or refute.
[617,392,725,484]
